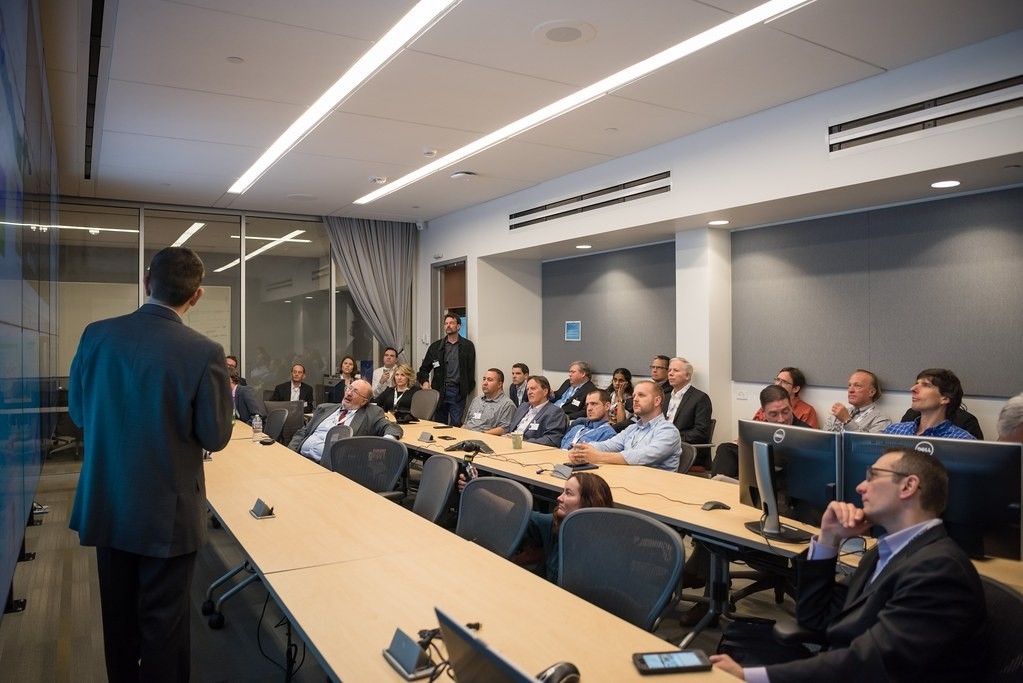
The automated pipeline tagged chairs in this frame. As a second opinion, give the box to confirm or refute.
[264,408,289,442]
[263,390,275,408]
[688,419,717,472]
[456,477,534,562]
[677,441,696,474]
[410,389,440,420]
[264,399,305,447]
[729,564,798,614]
[328,436,408,492]
[558,507,686,633]
[412,455,459,524]
[320,425,353,470]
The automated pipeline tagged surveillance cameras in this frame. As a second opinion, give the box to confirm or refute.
[415,222,428,230]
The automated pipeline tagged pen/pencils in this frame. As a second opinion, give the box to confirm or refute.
[433,426,453,428]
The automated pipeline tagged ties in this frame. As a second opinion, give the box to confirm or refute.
[339,409,348,427]
[293,388,299,401]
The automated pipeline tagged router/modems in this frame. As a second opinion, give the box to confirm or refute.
[451,172,479,182]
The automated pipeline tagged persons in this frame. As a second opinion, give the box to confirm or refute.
[327,355,359,395]
[457,467,615,586]
[732,367,1023,443]
[288,379,404,465]
[460,355,713,471]
[225,355,267,433]
[269,364,314,414]
[416,312,476,428]
[708,447,987,683]
[68,246,233,683]
[372,348,422,413]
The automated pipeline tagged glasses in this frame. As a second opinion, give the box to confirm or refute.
[773,377,792,387]
[649,365,667,370]
[866,466,922,491]
[347,386,365,399]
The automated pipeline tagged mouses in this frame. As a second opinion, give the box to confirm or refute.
[702,501,730,511]
[536,661,580,683]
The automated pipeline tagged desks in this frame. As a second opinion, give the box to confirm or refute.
[201,412,1023,683]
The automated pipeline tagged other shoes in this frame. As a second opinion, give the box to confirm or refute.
[681,573,706,590]
[679,606,720,628]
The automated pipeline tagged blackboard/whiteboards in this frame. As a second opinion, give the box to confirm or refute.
[0,279,57,378]
[57,281,230,377]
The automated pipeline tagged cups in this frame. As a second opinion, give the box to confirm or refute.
[370,402,377,406]
[511,433,523,448]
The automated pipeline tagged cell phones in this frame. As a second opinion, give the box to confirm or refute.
[259,439,275,445]
[438,436,455,440]
[632,647,712,673]
[433,425,453,428]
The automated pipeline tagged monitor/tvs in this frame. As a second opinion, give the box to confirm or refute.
[435,607,543,683]
[738,418,842,544]
[323,377,345,403]
[841,431,1023,562]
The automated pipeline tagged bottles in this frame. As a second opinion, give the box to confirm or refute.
[252,415,262,441]
[232,409,235,424]
